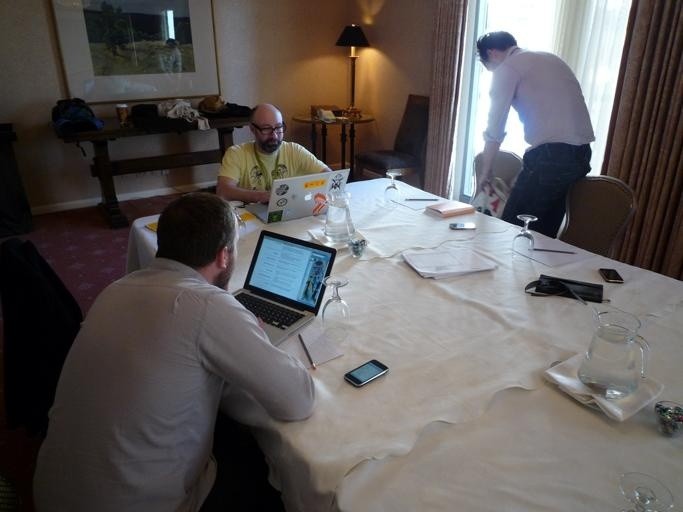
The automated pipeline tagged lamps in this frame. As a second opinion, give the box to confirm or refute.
[336,24,369,118]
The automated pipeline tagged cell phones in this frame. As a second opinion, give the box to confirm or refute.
[345,358,388,387]
[449,221,477,230]
[599,268,625,283]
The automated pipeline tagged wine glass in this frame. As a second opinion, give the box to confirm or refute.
[511,213,539,264]
[385,170,402,207]
[321,275,350,341]
[618,471,675,512]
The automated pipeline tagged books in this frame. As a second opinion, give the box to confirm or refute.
[426,200,474,218]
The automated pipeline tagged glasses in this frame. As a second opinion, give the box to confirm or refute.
[250,121,287,135]
[525,276,587,305]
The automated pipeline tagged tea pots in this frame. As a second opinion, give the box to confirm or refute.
[578,304,652,401]
[324,190,357,242]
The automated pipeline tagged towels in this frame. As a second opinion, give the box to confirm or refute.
[159,99,210,131]
[546,352,663,422]
[309,224,367,250]
[400,248,498,280]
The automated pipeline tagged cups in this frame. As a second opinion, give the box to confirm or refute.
[115,103,130,128]
[654,401,683,438]
[347,237,368,261]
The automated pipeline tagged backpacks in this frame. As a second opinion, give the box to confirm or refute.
[52,98,104,140]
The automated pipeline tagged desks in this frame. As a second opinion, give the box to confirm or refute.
[123,176,683,512]
[63,105,253,228]
[293,113,375,182]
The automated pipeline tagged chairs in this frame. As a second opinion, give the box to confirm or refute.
[468,150,523,218]
[560,175,637,258]
[1,239,83,436]
[356,94,429,189]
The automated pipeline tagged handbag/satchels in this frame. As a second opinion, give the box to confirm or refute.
[471,177,510,218]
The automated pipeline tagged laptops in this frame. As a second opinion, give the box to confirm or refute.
[245,169,351,225]
[231,229,336,348]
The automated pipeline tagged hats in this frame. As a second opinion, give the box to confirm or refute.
[199,95,225,113]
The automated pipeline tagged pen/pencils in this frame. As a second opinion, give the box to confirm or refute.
[406,198,438,200]
[534,249,576,254]
[298,334,316,369]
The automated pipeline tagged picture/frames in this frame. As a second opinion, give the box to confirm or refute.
[49,0,222,102]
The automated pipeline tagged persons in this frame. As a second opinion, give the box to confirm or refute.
[214,103,333,205]
[475,31,595,239]
[32,193,316,512]
[149,38,182,91]
